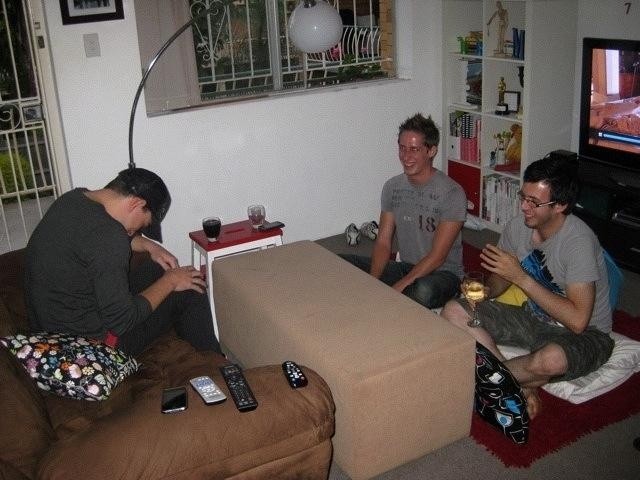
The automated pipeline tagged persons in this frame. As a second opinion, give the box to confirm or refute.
[496,77,506,105]
[338,111,468,309]
[439,154,616,420]
[22,167,228,361]
[506,124,522,160]
[486,1,509,53]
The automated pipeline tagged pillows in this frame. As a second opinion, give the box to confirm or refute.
[0,330,141,400]
[474,341,532,447]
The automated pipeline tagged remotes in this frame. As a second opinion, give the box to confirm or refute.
[282,360,308,389]
[189,375,228,406]
[221,364,258,412]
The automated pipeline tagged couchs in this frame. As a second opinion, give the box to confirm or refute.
[0,250,334,480]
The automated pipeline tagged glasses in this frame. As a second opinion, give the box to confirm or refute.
[515,189,559,210]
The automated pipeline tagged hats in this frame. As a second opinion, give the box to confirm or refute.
[118,167,171,244]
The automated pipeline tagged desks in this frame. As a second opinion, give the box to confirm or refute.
[189,220,283,343]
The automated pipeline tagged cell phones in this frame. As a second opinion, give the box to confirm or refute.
[258,221,285,231]
[161,386,187,414]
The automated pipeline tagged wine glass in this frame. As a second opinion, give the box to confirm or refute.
[462,271,484,326]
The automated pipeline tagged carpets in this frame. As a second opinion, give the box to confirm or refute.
[389,239,639,468]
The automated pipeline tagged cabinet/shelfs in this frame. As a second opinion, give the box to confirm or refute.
[574,157,640,276]
[442,0,579,234]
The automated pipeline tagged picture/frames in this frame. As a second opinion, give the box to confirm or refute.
[58,1,124,26]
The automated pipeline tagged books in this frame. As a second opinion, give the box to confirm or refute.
[512,27,525,57]
[482,172,519,229]
[448,29,483,165]
[517,30,525,60]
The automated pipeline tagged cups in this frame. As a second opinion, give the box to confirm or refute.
[201,216,223,242]
[247,205,266,229]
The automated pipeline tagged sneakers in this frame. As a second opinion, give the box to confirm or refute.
[361,221,379,240]
[345,223,361,246]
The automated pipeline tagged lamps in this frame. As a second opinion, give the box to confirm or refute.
[129,1,343,169]
